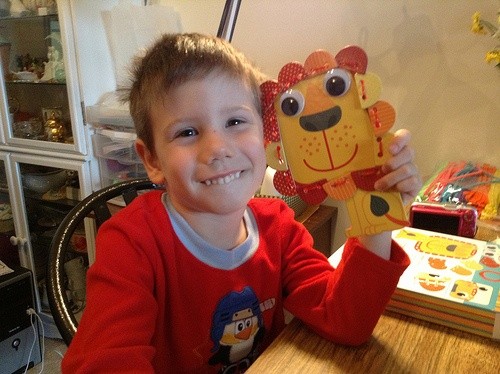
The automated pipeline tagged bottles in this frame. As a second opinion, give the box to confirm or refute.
[63,257,86,302]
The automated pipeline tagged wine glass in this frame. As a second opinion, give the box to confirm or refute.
[14,117,44,140]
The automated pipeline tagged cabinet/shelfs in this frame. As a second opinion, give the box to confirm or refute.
[84,105,147,188]
[0,0,116,340]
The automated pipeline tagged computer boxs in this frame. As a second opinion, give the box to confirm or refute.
[0,262,42,374]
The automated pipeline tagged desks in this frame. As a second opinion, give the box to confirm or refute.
[242,219,500,374]
[304,205,337,258]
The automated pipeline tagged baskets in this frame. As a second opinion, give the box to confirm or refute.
[254,192,307,220]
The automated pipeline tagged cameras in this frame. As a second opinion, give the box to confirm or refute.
[409,204,477,239]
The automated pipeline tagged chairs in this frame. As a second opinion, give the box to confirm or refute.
[46,177,153,346]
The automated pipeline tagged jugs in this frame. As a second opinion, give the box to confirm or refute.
[9,0,28,15]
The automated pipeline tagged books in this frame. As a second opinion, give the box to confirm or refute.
[327,227,500,341]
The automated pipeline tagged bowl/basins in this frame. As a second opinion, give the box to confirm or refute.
[20,165,67,193]
[12,70,37,80]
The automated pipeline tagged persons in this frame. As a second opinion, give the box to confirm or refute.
[59,32,421,374]
